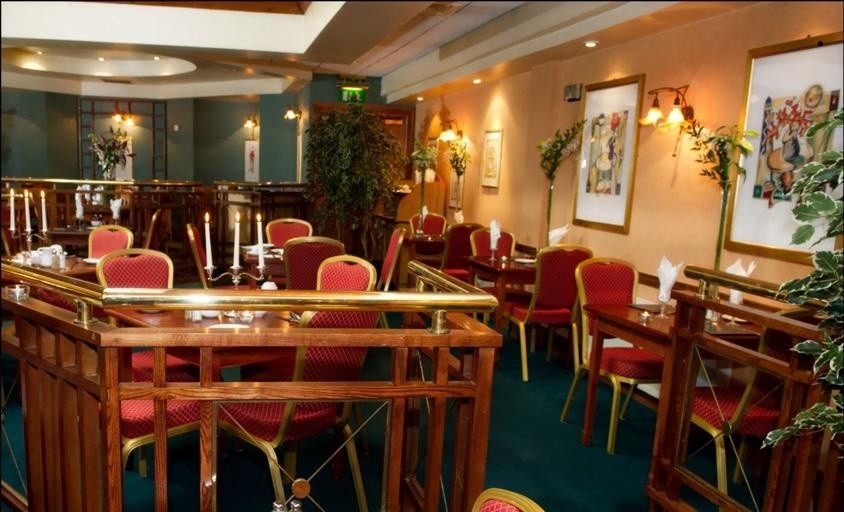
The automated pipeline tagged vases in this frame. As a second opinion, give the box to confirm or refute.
[103,164,116,180]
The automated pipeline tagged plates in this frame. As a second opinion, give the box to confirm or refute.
[515,258,537,263]
[82,258,100,265]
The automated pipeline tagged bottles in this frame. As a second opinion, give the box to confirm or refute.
[59,251,66,269]
[51,250,59,269]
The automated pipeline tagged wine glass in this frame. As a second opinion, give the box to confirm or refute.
[654,288,671,320]
[725,293,743,327]
[488,241,498,261]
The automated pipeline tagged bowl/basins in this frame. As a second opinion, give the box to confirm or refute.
[5,284,30,300]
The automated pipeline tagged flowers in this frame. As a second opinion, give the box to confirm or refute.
[86,127,137,174]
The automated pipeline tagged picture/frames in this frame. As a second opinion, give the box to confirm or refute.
[479,129,504,189]
[572,73,645,235]
[723,30,843,267]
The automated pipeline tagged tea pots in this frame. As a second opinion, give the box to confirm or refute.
[37,247,57,268]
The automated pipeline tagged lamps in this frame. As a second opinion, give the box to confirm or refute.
[638,83,694,132]
[283,104,302,120]
[244,111,257,128]
[437,116,463,144]
